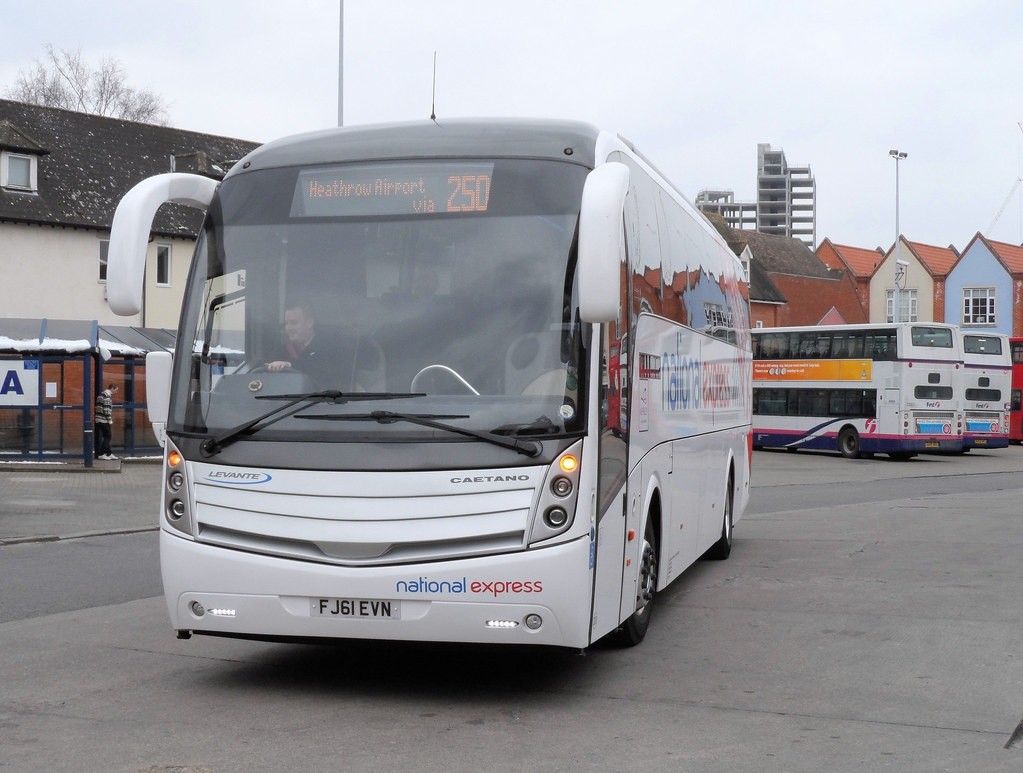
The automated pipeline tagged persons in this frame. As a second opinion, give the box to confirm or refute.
[265,301,329,381]
[93,383,120,461]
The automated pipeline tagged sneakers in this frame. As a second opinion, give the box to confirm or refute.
[107,453,118,459]
[98,454,111,460]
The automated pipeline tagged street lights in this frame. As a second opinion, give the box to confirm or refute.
[889,149,909,323]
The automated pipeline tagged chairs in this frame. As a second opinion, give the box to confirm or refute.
[502,329,584,400]
[270,320,359,395]
[752,345,897,417]
[358,335,391,392]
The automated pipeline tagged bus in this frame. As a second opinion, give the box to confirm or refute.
[1008,337,1023,446]
[749,319,1013,458]
[103,117,753,658]
